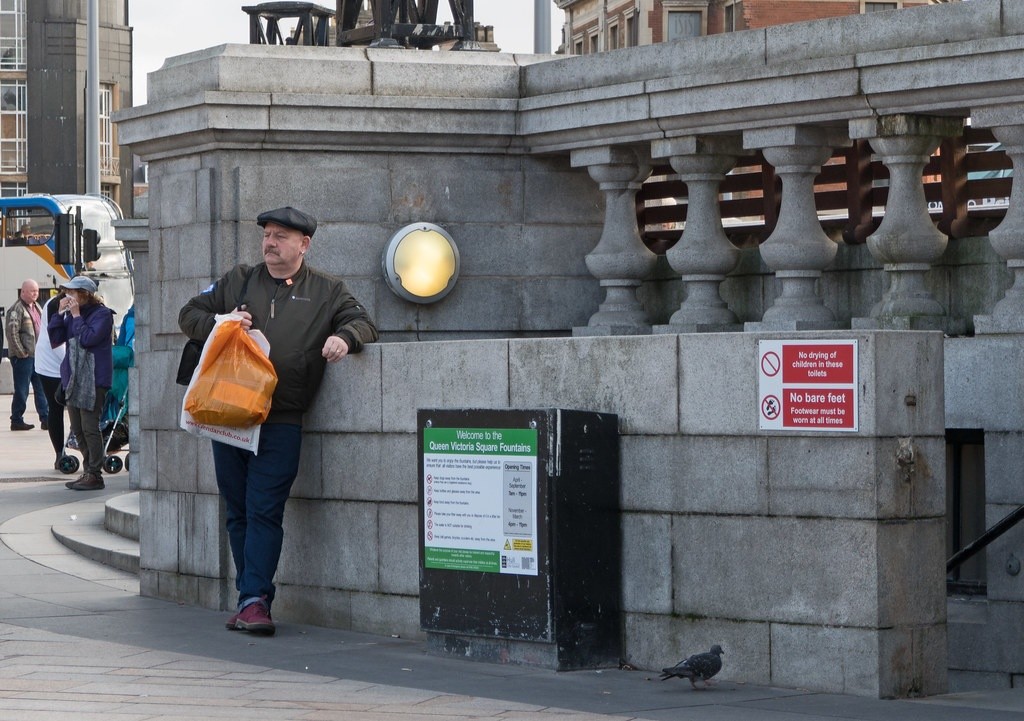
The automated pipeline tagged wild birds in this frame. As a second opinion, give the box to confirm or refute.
[660,644,725,689]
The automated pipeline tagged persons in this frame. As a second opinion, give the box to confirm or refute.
[179,207,380,637]
[17,224,33,239]
[5,276,113,491]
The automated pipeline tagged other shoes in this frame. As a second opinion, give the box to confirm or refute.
[41,423,48,430]
[72,473,105,490]
[55,452,66,470]
[65,474,84,489]
[235,594,275,636]
[225,613,238,628]
[11,421,34,431]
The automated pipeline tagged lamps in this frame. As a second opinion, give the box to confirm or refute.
[381,222,461,305]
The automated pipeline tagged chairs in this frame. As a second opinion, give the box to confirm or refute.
[25,234,50,245]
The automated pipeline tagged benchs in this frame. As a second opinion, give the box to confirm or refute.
[241,1,336,46]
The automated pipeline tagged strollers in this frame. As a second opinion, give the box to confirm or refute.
[56,344,136,475]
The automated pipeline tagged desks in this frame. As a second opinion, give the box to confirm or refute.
[335,0,475,48]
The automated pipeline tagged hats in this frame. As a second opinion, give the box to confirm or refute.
[59,276,97,294]
[257,207,317,236]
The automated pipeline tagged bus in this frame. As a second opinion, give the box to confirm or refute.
[0,192,135,360]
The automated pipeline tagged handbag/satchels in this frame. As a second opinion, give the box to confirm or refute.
[176,339,203,386]
[180,314,271,456]
[184,319,278,428]
